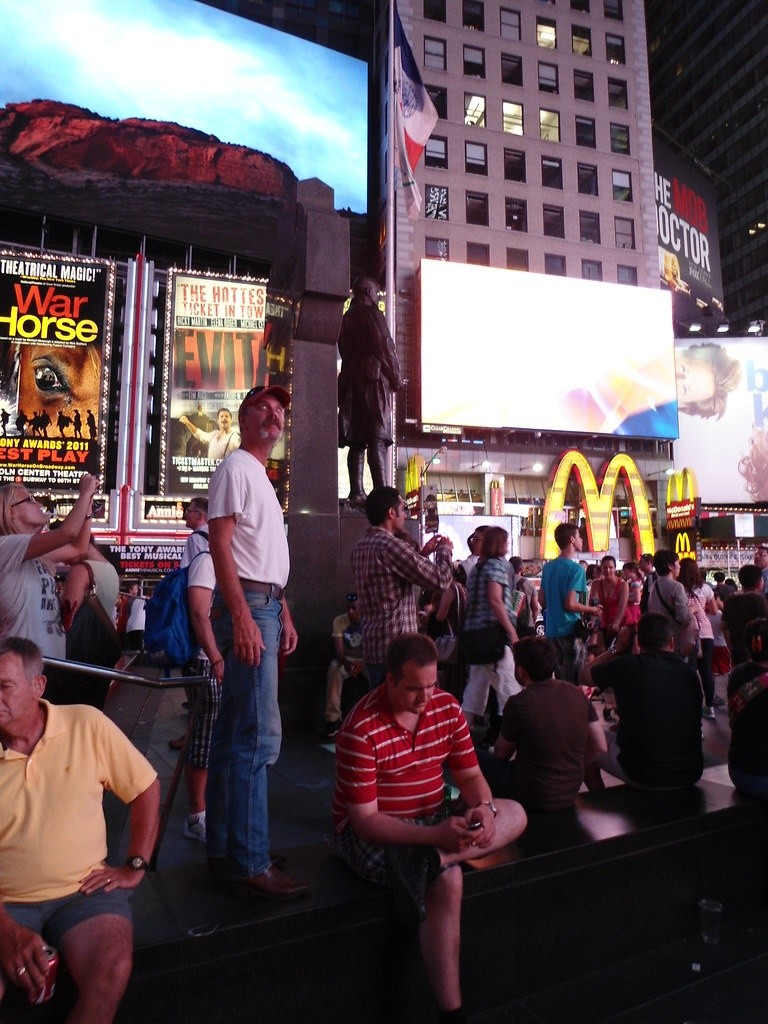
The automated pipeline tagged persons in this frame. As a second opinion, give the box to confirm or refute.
[677,342,742,422]
[351,486,454,686]
[206,387,311,902]
[180,497,224,841]
[417,523,768,811]
[320,593,369,737]
[0,473,150,706]
[331,633,528,1024]
[338,277,404,500]
[0,636,160,1024]
[179,404,241,458]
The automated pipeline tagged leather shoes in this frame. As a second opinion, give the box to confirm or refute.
[226,859,309,903]
[208,851,286,880]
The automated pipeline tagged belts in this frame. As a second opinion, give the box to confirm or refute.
[239,577,284,601]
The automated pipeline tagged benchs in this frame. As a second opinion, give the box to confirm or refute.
[3,761,744,994]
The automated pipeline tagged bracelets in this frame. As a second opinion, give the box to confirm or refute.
[85,513,94,520]
[608,647,618,655]
[476,801,497,818]
[212,659,223,665]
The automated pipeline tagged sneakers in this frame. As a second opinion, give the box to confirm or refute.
[702,707,715,718]
[182,817,207,843]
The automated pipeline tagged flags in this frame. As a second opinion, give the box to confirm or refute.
[394,4,438,215]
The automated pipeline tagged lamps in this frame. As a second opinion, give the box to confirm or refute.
[648,468,675,477]
[425,458,441,465]
[471,461,491,469]
[520,463,544,472]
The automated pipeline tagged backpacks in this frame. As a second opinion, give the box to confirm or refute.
[142,550,212,666]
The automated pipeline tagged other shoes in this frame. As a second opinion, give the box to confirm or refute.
[168,737,186,750]
[713,695,725,705]
[324,720,341,738]
[182,702,189,709]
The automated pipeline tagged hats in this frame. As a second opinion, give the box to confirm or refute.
[55,571,67,580]
[240,386,293,413]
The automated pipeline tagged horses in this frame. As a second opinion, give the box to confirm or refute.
[5,339,101,438]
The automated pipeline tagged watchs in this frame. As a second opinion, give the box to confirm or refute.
[126,854,149,872]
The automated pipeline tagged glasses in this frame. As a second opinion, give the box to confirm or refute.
[10,495,36,506]
[186,509,199,515]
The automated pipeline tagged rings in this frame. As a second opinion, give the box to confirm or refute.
[621,627,623,630]
[106,877,110,883]
[16,967,25,975]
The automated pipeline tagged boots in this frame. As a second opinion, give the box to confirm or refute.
[392,844,441,921]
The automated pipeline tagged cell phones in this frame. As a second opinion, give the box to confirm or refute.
[468,822,482,830]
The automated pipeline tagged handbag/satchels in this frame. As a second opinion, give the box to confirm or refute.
[463,635,505,664]
[65,561,124,667]
[680,620,702,659]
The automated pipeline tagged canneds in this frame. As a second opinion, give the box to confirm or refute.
[28,946,58,1003]
